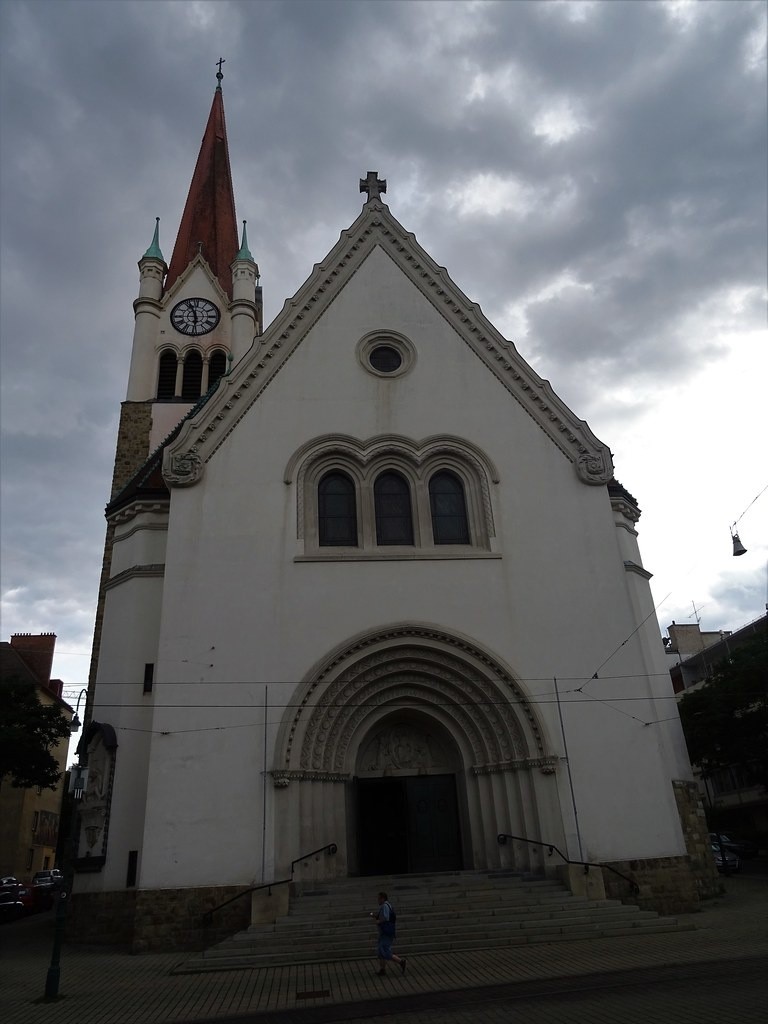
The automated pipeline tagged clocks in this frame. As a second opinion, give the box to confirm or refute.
[170,297,221,336]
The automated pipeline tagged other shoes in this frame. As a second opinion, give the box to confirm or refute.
[399,959,406,975]
[375,969,385,976]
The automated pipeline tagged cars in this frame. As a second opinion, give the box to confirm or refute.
[711,840,742,873]
[0,868,64,919]
[709,832,746,857]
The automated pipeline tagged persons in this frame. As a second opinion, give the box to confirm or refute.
[369,892,406,976]
